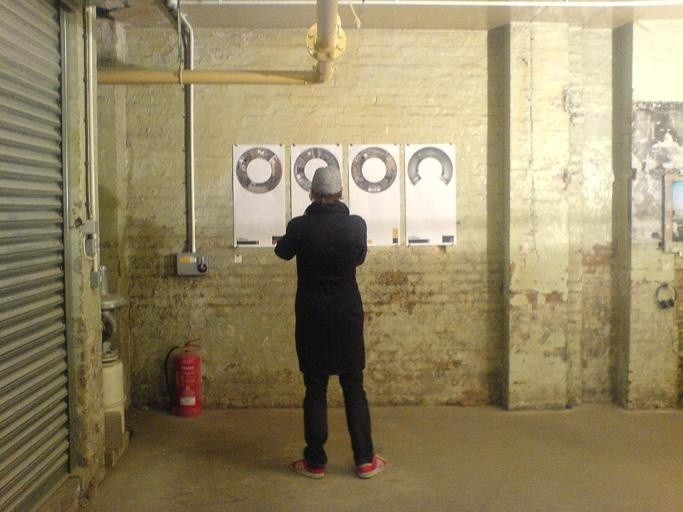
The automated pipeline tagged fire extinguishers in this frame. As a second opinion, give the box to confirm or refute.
[164,337,203,415]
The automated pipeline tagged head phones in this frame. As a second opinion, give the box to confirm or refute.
[655,283,677,310]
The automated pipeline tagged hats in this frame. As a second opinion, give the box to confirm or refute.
[312,167,342,195]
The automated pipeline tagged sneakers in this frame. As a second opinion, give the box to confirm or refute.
[289,459,325,479]
[357,453,388,478]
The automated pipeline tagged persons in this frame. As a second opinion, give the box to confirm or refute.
[273,165,388,479]
